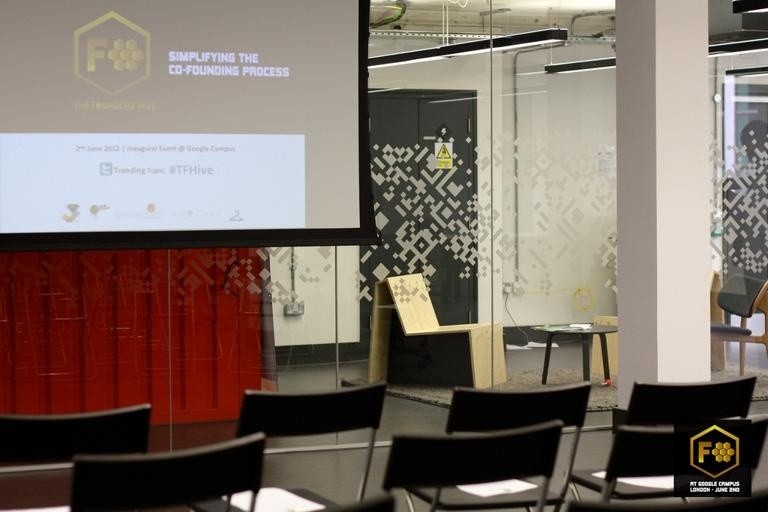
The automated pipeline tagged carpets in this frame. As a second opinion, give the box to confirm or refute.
[341,361,767,412]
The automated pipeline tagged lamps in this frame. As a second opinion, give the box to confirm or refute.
[707,31,768,57]
[368,28,569,70]
[725,65,768,76]
[544,55,615,74]
[732,0,768,14]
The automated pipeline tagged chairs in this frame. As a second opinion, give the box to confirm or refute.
[188,379,388,512]
[313,494,397,512]
[401,381,591,512]
[367,272,506,390]
[710,273,768,376]
[563,375,757,512]
[601,414,768,501]
[381,420,563,511]
[70,431,266,512]
[1,403,152,467]
[566,489,768,512]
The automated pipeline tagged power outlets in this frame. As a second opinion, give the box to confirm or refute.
[502,283,514,294]
[513,280,526,295]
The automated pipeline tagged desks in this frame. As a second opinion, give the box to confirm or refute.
[530,324,618,385]
